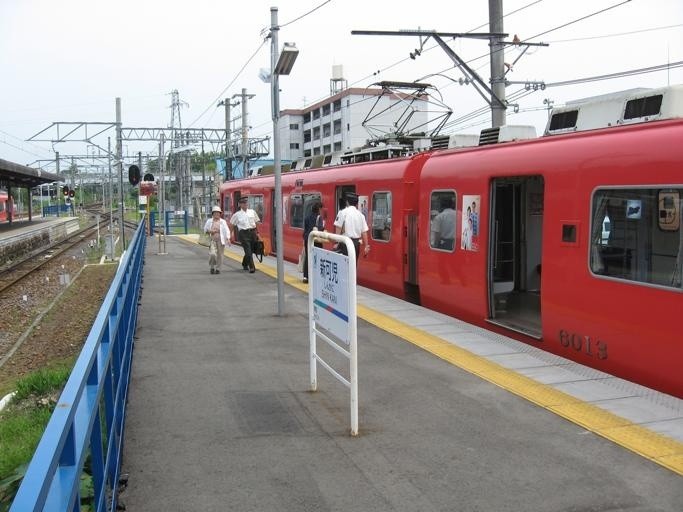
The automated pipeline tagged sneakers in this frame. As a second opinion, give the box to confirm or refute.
[242,263,255,273]
[210,269,221,275]
[303,279,308,283]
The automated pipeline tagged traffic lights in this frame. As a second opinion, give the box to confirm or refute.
[129,164,140,186]
[63,187,75,197]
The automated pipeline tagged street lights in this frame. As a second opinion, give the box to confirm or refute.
[259,44,299,317]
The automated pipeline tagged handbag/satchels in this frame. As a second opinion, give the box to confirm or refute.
[198,233,212,247]
[252,240,264,263]
[298,242,305,273]
[313,216,330,243]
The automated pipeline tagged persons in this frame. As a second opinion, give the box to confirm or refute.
[302,191,370,285]
[430,195,456,248]
[203,196,262,274]
[464,201,479,251]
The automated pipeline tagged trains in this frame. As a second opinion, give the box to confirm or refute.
[217,87,682,400]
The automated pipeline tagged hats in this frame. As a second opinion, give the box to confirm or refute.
[211,206,223,214]
[346,191,360,200]
[238,196,249,203]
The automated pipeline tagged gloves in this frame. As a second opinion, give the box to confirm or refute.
[363,247,369,258]
[332,241,340,250]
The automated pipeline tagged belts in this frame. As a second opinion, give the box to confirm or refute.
[242,229,257,232]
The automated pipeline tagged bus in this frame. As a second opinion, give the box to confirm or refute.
[32,182,79,206]
[0,191,14,223]
